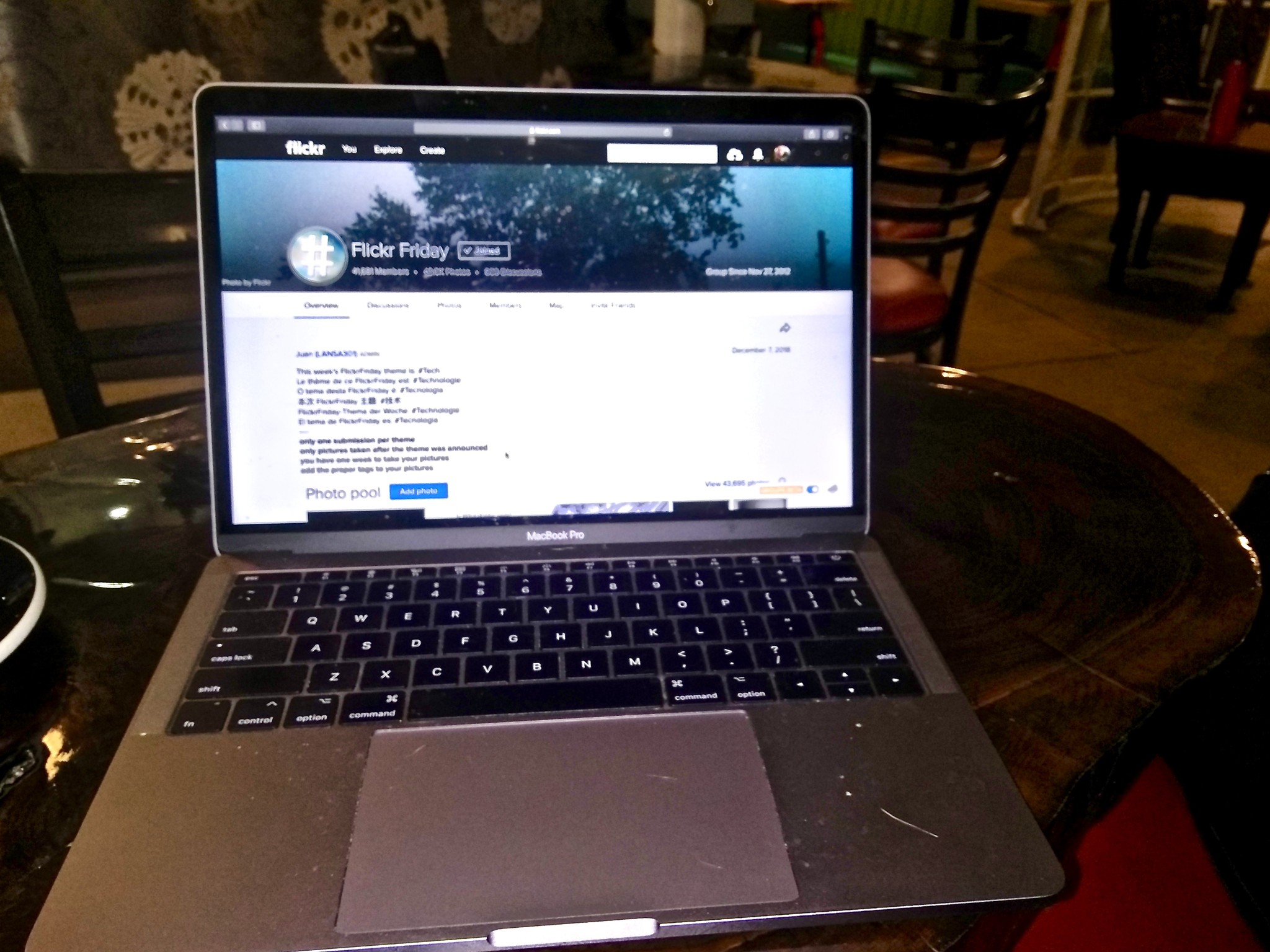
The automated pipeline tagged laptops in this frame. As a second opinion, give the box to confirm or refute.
[27,82,1070,952]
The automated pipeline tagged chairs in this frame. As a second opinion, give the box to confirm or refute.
[961,466,1270,952]
[0,155,205,437]
[702,15,1045,370]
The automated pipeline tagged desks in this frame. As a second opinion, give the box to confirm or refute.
[1,363,1264,951]
[1104,110,1270,312]
